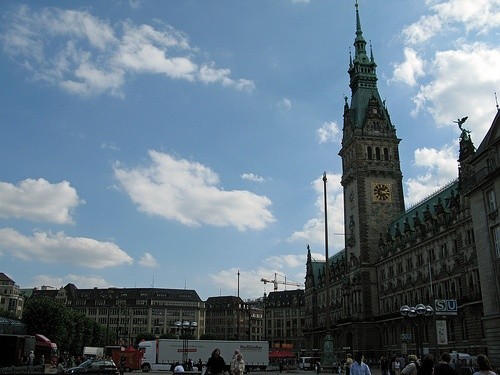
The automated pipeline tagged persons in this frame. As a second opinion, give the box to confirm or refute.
[15,348,127,375]
[170,349,245,375]
[270,359,300,373]
[308,351,499,375]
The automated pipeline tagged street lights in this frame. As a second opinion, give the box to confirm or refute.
[400,304,434,359]
[174,321,198,371]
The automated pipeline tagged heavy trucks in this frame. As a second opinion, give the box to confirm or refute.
[138,339,269,372]
[0,334,57,368]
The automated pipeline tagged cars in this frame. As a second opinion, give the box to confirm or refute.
[64,360,119,375]
[317,358,341,375]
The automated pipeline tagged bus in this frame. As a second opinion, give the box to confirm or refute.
[299,357,321,371]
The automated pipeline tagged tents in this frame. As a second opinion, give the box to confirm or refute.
[269,351,295,363]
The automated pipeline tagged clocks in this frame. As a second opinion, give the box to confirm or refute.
[370,181,392,204]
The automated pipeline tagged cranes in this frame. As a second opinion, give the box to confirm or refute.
[261,272,305,291]
